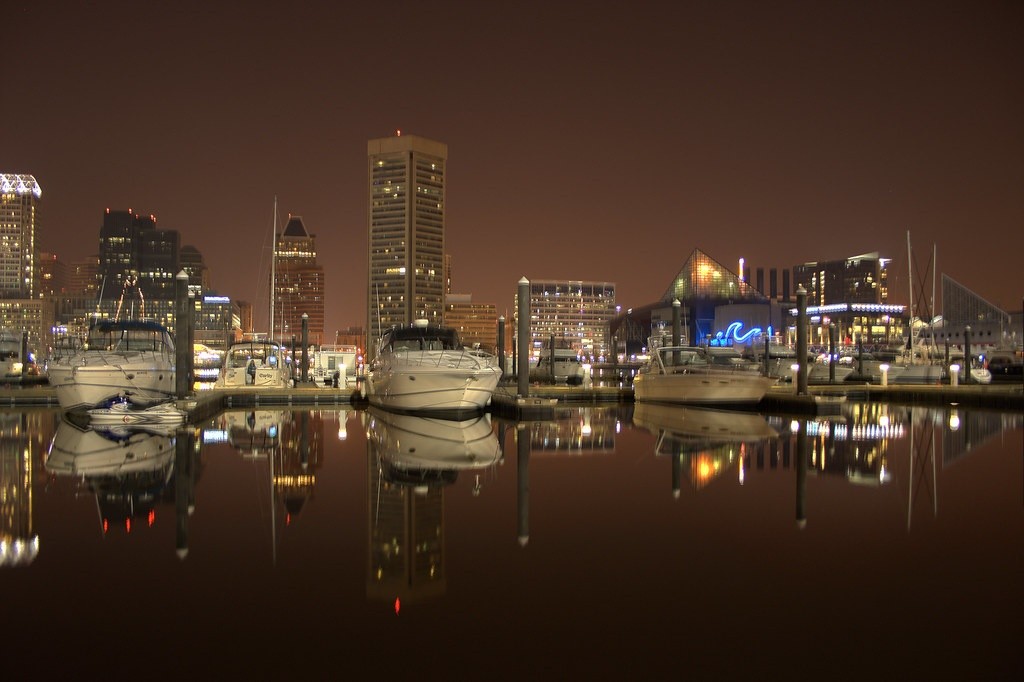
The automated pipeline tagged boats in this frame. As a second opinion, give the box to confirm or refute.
[357,401,503,525]
[43,405,176,543]
[45,276,179,411]
[214,194,362,389]
[0,327,49,385]
[633,334,782,409]
[530,323,588,383]
[629,401,782,457]
[354,283,504,414]
[740,229,995,385]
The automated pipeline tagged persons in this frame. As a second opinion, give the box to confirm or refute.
[262,357,264,365]
[247,360,256,384]
[28,348,40,375]
